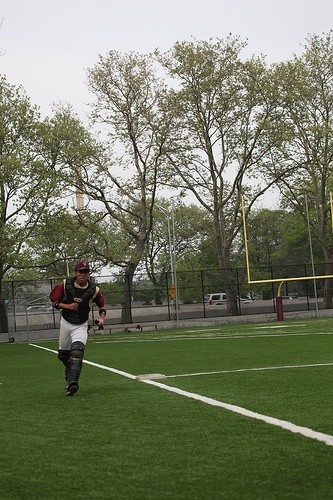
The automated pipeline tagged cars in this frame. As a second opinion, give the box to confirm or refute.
[274,296,298,299]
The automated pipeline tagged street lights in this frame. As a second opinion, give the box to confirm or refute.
[149,192,189,309]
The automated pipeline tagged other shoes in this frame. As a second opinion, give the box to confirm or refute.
[64,382,69,389]
[66,382,79,396]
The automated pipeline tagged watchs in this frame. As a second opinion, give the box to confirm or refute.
[54,301,60,308]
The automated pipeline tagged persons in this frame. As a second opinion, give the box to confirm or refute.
[48,259,107,396]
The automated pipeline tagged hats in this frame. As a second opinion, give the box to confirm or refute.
[75,261,90,272]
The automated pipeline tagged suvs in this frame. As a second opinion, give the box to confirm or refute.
[206,293,241,306]
